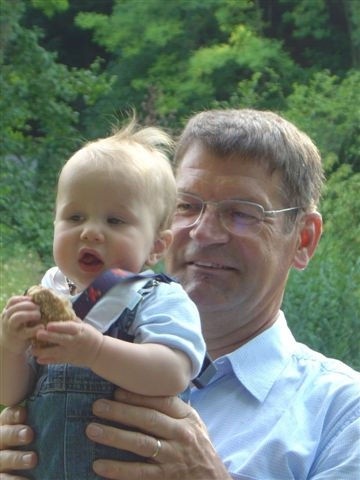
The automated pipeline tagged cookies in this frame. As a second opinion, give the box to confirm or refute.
[24,284,78,358]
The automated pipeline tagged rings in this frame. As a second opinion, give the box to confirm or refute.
[152,439,162,459]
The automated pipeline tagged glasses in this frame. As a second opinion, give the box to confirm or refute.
[171,192,317,236]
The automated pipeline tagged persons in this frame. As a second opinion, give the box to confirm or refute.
[3,131,232,479]
[1,109,360,479]
